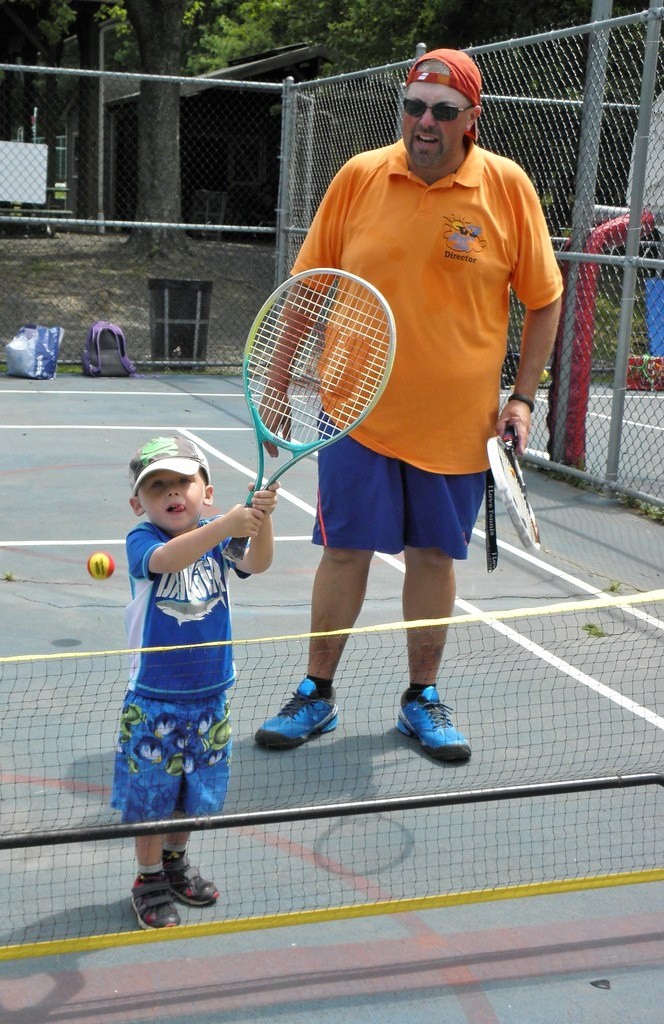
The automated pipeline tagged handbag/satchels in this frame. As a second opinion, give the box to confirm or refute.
[4,323,66,380]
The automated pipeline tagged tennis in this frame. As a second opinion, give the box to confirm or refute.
[87,551,114,580]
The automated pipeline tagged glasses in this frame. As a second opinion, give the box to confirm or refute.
[401,98,473,122]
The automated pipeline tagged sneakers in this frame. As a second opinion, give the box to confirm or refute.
[129,878,180,929]
[164,858,218,906]
[396,686,472,761]
[254,678,338,749]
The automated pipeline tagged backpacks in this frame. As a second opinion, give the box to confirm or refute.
[82,323,135,377]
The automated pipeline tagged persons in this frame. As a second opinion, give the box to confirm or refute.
[110,434,280,929]
[254,49,564,762]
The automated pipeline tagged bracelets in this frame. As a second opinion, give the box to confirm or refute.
[508,394,534,413]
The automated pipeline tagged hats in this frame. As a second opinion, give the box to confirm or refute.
[407,48,482,141]
[130,438,211,496]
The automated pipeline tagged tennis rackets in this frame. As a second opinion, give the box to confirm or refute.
[221,266,398,565]
[486,423,542,556]
